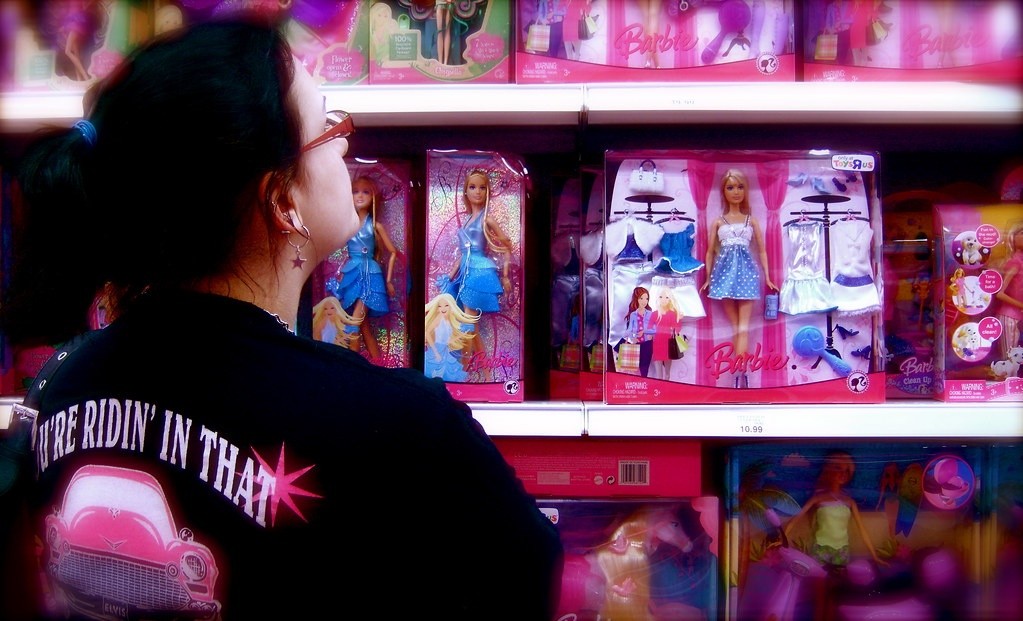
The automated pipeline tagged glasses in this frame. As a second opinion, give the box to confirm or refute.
[299,109,357,154]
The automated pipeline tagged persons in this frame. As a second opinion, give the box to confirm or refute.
[782,450,890,621]
[324,175,396,366]
[435,0,454,65]
[595,504,707,621]
[46,0,94,81]
[435,170,512,384]
[996,228,1023,362]
[698,168,781,389]
[936,1,957,69]
[0,12,563,621]
[638,0,663,69]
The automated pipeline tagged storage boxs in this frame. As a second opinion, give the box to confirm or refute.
[0,0,1023,620]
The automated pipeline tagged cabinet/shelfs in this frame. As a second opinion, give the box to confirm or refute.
[0,82,1023,440]
